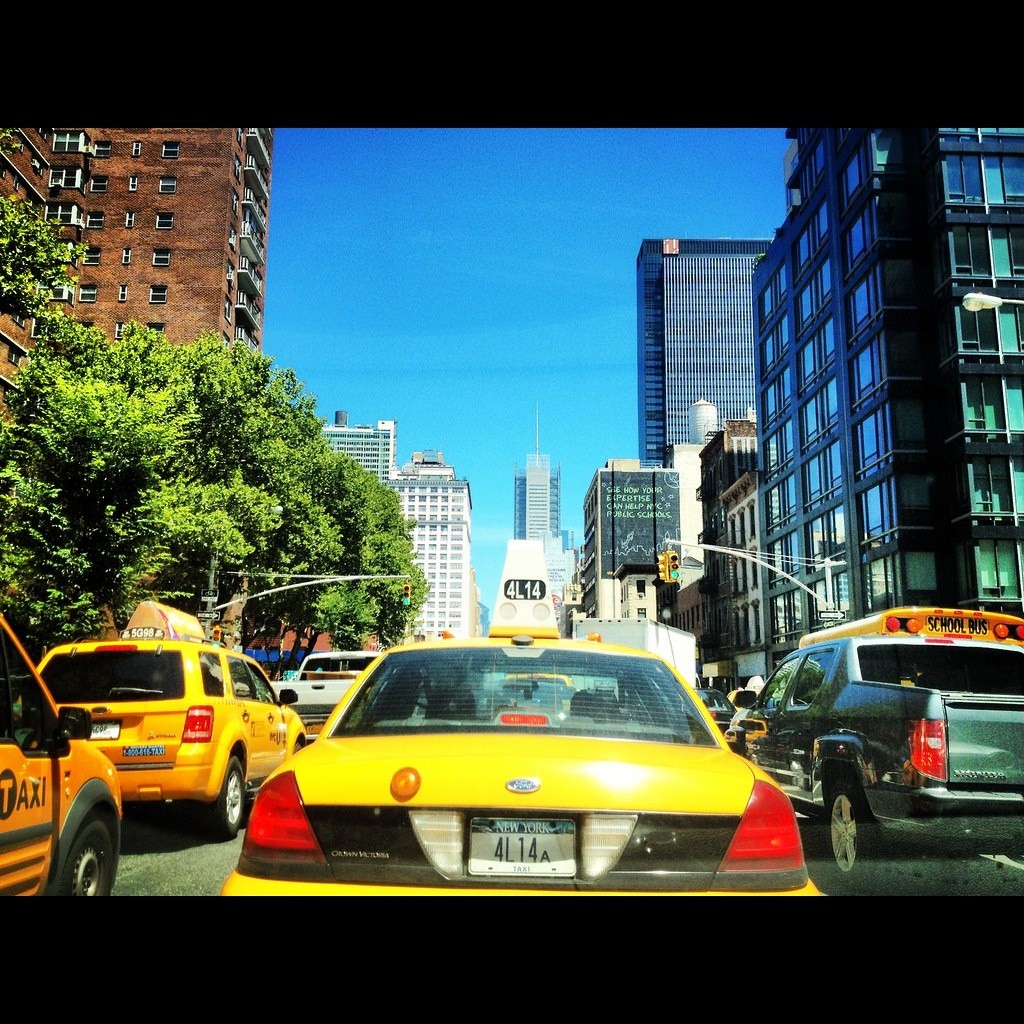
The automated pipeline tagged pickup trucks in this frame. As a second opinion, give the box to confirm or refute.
[266,651,387,741]
[725,634,1024,886]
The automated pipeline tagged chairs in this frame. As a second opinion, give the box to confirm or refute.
[424,688,476,718]
[570,690,622,722]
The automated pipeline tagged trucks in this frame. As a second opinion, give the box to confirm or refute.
[570,617,701,700]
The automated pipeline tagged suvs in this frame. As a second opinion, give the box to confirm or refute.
[222,539,829,896]
[0,612,124,895]
[12,601,308,838]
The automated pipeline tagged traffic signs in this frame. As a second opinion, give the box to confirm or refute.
[197,611,220,621]
[819,610,846,621]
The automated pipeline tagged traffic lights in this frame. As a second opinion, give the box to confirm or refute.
[212,626,221,643]
[402,584,411,606]
[655,554,666,581]
[667,550,683,584]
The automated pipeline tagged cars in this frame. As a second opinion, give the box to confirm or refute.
[725,676,766,711]
[694,688,738,739]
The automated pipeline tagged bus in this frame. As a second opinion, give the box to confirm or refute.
[798,605,1024,649]
